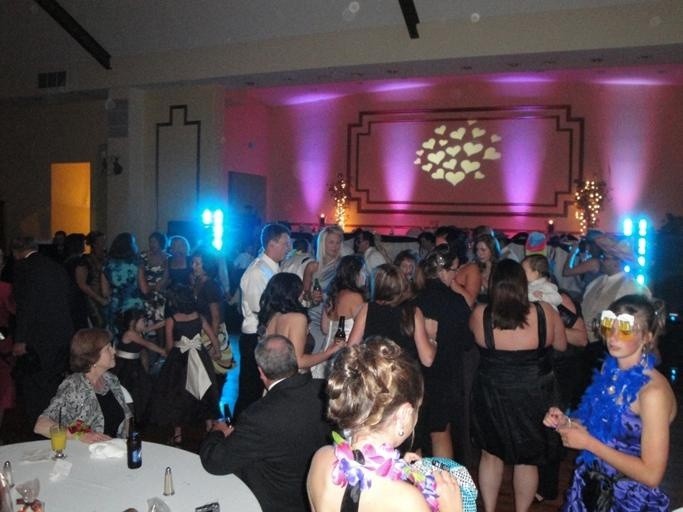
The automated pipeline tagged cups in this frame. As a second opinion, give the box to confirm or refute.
[13,479,39,503]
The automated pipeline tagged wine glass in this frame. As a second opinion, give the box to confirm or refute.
[47,410,68,461]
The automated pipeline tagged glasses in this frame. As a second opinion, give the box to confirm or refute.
[443,266,461,274]
[598,320,645,339]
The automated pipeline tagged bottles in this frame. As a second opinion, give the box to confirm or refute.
[125,417,142,468]
[223,404,233,428]
[163,467,174,495]
[312,278,321,306]
[2,461,14,488]
[333,315,345,344]
